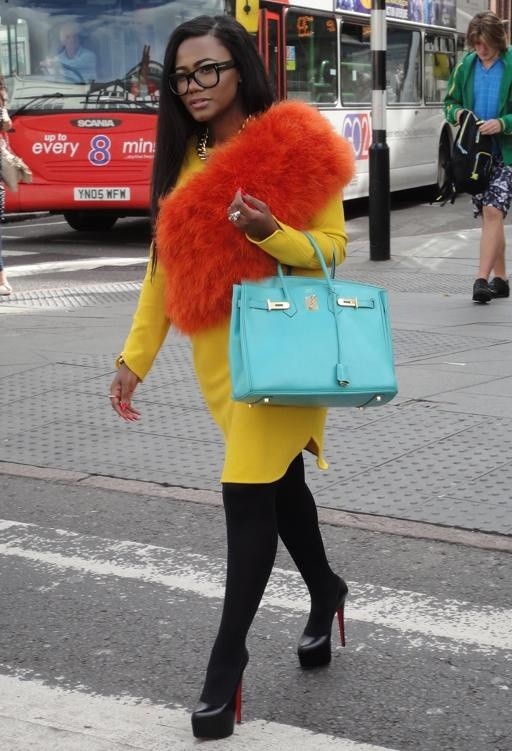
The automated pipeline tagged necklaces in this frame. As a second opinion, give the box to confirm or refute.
[196,113,254,163]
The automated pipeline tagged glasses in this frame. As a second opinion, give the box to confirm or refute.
[168,58,235,96]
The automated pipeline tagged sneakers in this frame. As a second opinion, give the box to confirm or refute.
[472,277,509,301]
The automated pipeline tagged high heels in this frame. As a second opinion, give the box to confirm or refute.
[299,576,348,668]
[191,646,249,739]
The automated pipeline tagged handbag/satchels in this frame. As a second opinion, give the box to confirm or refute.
[227,274,397,408]
[450,110,495,195]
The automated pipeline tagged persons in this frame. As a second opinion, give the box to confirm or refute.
[443,12,512,303]
[40,21,97,84]
[0,74,33,297]
[108,14,357,740]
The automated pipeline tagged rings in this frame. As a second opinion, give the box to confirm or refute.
[227,211,241,225]
[108,394,121,399]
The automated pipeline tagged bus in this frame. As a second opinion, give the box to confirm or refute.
[0,0,477,229]
